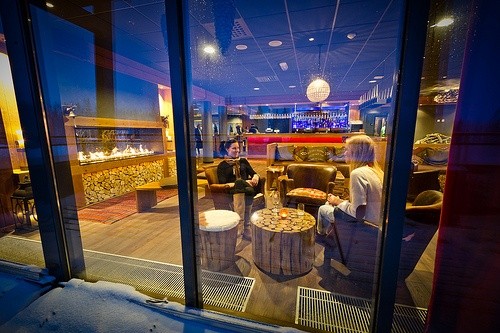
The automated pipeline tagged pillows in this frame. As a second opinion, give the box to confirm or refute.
[425,147,448,166]
[276,144,345,162]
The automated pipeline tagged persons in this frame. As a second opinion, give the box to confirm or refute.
[249,124,258,133]
[316,135,385,244]
[216,139,263,240]
[195,124,202,150]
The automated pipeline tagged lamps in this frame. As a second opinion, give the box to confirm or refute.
[305,45,330,103]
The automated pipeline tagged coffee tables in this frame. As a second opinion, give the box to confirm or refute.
[409,169,442,201]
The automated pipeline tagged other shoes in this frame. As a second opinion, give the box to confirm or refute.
[314,232,328,242]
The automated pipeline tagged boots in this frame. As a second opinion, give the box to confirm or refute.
[233,193,245,237]
[244,203,253,240]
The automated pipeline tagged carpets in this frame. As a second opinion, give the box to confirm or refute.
[76,188,178,224]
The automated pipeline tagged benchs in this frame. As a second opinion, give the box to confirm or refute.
[135,180,206,212]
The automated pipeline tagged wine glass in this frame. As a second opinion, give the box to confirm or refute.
[272,191,281,212]
[297,202,306,224]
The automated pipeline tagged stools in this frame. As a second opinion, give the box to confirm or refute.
[199,209,240,271]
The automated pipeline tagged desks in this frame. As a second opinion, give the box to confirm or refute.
[250,207,316,276]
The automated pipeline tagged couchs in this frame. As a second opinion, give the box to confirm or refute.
[278,163,337,216]
[205,164,266,221]
[412,143,450,187]
[266,143,351,191]
[322,190,442,293]
